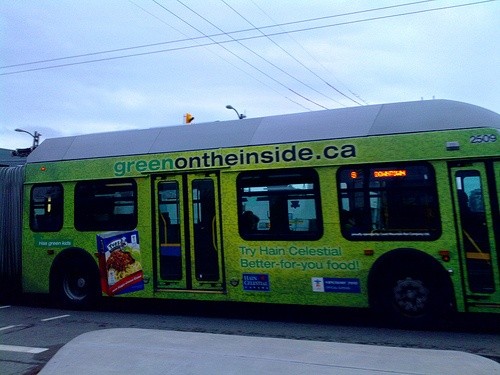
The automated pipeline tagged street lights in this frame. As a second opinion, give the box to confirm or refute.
[15,128,38,148]
[225,105,246,119]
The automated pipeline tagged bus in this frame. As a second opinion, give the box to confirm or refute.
[0,97,500,330]
